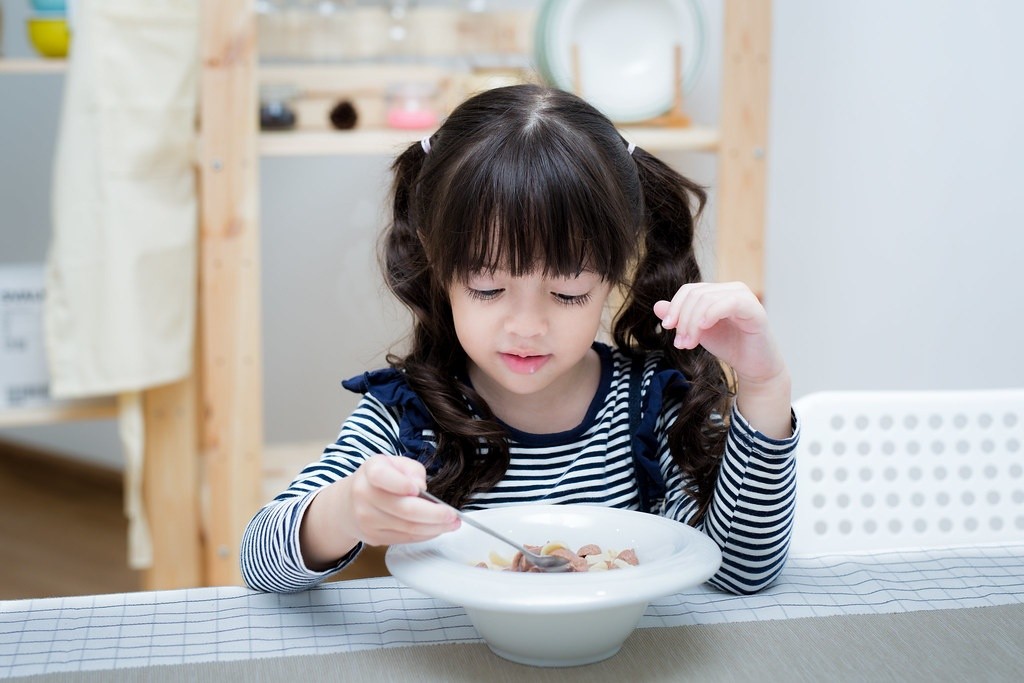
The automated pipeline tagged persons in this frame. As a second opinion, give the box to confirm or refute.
[240,85,802,597]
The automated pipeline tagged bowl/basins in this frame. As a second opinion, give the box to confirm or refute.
[387,93,439,131]
[25,21,71,58]
[385,505,722,667]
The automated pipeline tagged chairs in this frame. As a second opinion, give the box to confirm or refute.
[795,391,1024,546]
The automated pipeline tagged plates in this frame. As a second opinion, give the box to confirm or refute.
[534,0,709,124]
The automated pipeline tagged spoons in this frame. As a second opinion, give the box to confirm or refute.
[418,490,573,573]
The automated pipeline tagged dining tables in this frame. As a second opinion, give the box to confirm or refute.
[0,543,1024,683]
